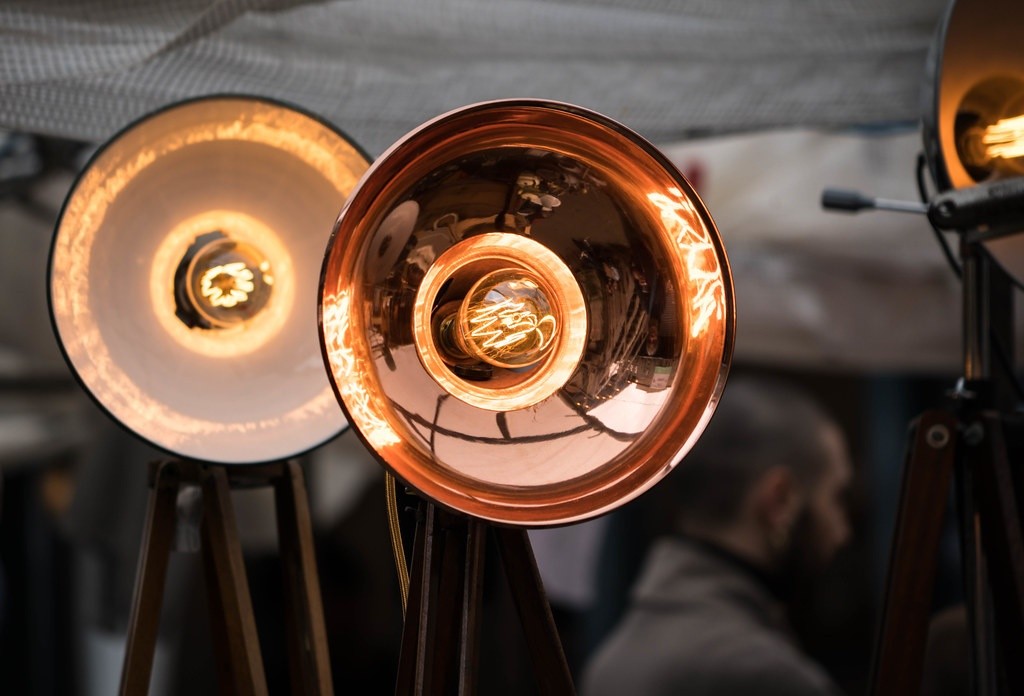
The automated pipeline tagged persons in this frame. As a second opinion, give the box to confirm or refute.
[570,193,665,356]
[583,388,849,695]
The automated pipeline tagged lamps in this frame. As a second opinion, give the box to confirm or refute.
[918,0,1024,292]
[315,98,738,533]
[41,96,375,466]
